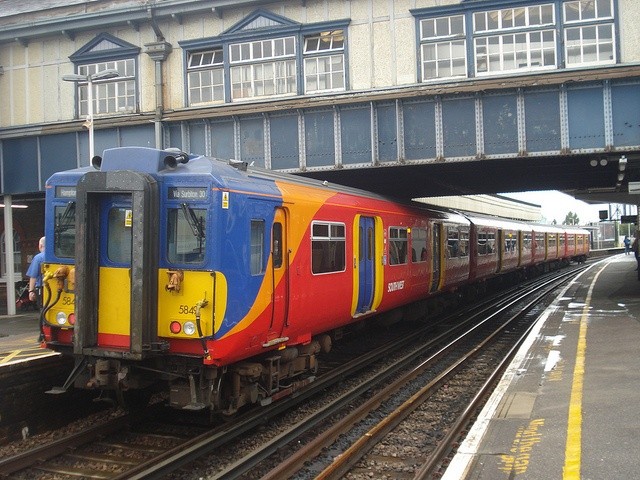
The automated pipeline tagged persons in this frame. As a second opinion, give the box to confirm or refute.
[623,235,631,255]
[26,236,45,342]
